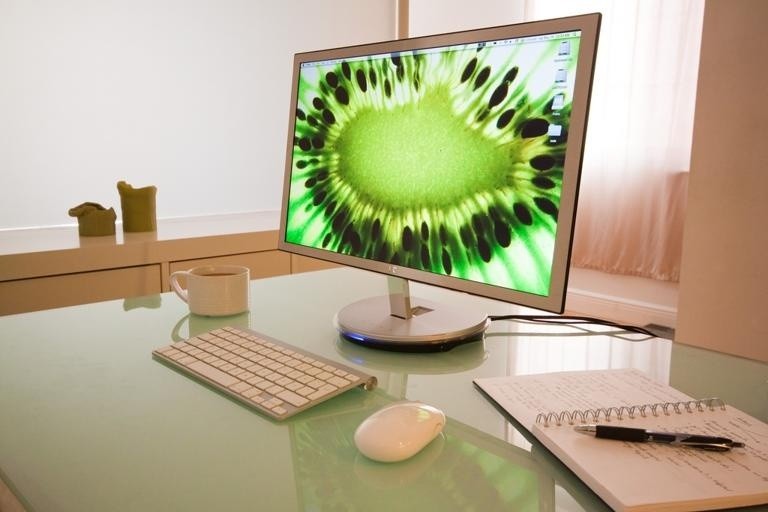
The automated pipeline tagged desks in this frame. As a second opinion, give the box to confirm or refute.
[1,267,768,508]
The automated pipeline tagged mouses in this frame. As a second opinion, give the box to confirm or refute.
[353,400,447,463]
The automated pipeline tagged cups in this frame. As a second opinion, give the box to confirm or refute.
[170,264,248,316]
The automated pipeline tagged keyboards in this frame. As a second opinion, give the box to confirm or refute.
[150,323,379,422]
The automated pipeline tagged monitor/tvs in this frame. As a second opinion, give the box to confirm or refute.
[276,11,602,353]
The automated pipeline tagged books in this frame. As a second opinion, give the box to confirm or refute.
[472,364,768,512]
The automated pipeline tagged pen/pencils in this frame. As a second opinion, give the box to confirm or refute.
[574,425,745,452]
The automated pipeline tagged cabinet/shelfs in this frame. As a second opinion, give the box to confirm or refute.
[0,212,332,315]
